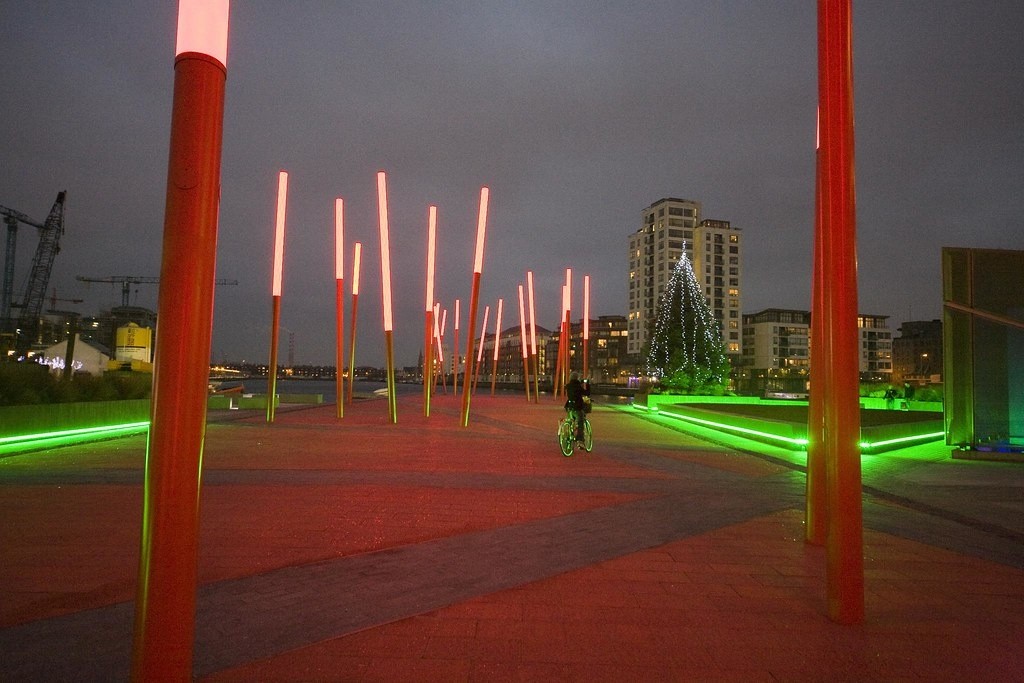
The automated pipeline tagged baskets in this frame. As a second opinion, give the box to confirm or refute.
[583,399,592,413]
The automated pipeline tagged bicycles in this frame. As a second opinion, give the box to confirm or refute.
[555,399,595,457]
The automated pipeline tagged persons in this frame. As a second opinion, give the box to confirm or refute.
[565,371,591,450]
[883,385,898,413]
[904,381,915,410]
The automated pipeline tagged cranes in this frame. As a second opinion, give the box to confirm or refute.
[0,190,83,361]
[76,271,238,307]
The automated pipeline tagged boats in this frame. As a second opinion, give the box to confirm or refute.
[212,382,246,395]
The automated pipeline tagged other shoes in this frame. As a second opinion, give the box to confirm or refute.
[577,440,586,449]
